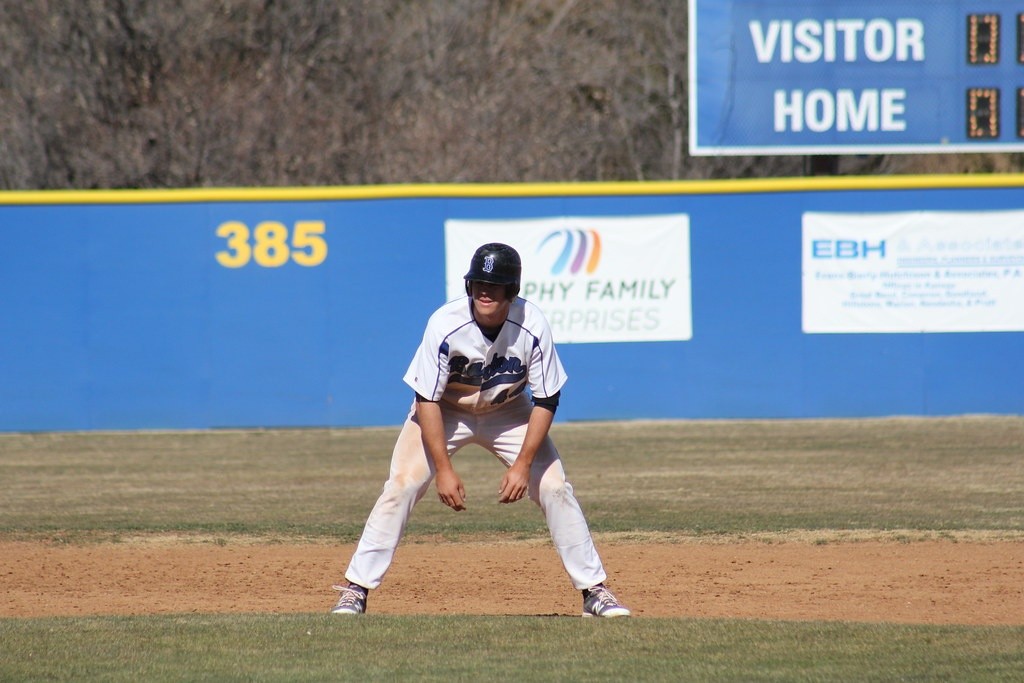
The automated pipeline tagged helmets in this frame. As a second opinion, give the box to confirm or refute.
[463,243,522,303]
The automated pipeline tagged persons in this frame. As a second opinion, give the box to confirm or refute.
[331,242,632,619]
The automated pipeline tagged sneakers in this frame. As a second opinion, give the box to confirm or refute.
[332,581,369,614]
[581,585,630,618]
